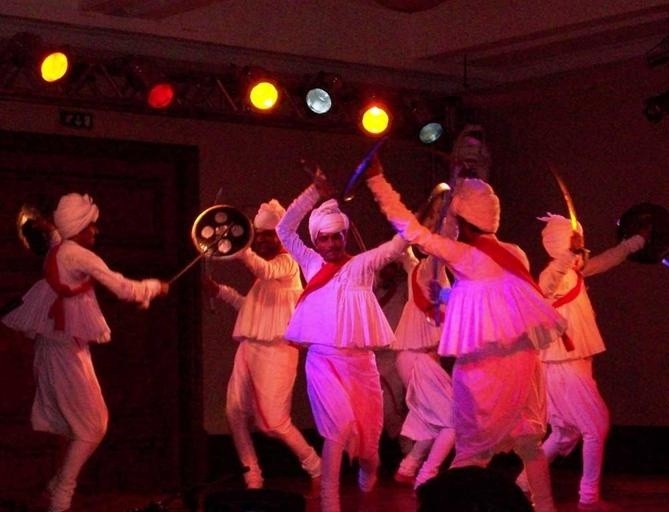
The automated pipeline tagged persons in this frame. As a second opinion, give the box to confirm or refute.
[416,465,532,512]
[367,156,569,512]
[3,194,169,512]
[275,171,423,512]
[514,215,648,510]
[207,199,323,497]
[204,489,304,512]
[374,192,461,488]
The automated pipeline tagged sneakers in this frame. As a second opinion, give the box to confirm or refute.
[303,476,324,500]
[393,472,416,486]
[578,498,618,512]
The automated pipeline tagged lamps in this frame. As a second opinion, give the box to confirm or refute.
[406,103,444,147]
[305,70,344,117]
